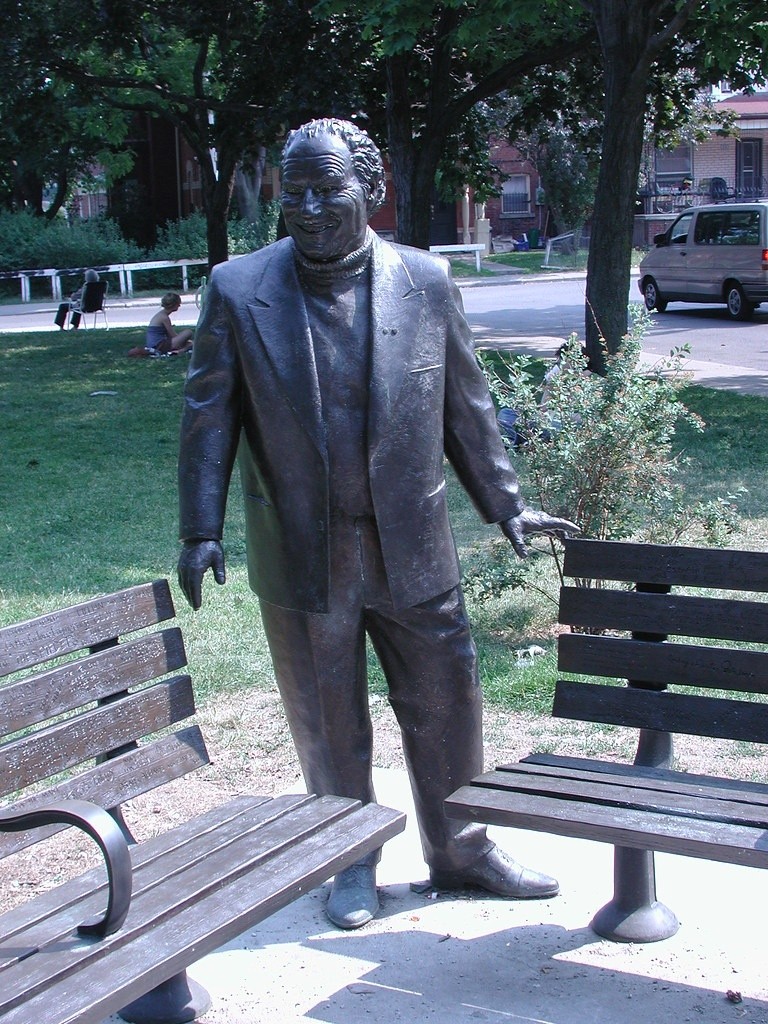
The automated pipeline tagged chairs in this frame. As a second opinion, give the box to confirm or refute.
[710,177,737,204]
[67,280,109,334]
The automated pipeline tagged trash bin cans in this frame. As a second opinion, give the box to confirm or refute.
[528,229,540,249]
[513,241,529,252]
[538,237,547,249]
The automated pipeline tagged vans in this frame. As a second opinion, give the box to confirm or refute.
[637,200,768,322]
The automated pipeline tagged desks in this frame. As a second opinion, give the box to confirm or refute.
[644,190,711,213]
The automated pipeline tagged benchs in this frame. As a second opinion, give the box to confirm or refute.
[0,577,407,1024]
[439,537,768,943]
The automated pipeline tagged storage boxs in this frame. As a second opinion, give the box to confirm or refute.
[514,239,530,252]
[538,236,550,248]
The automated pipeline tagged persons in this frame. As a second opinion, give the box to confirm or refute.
[146,293,193,355]
[491,343,608,454]
[52,269,100,332]
[179,117,582,929]
